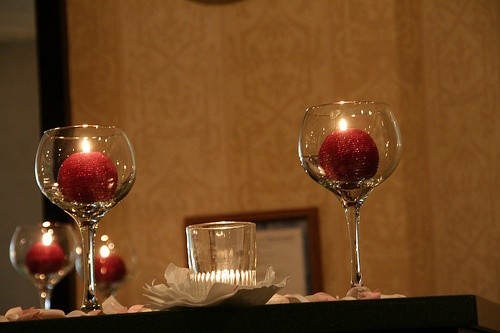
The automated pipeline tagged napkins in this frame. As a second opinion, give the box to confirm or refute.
[143,262,294,308]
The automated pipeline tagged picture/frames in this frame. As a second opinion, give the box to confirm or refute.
[184,204,324,295]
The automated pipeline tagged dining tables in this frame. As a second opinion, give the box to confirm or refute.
[0,295,500,333]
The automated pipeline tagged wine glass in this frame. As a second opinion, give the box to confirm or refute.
[10,222,79,311]
[297,101,402,288]
[35,125,137,312]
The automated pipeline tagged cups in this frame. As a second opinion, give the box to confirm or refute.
[186,221,257,287]
[76,234,133,300]
